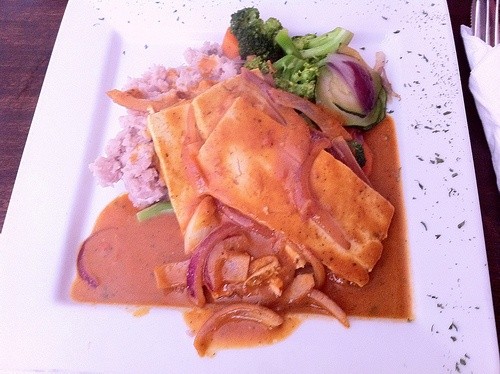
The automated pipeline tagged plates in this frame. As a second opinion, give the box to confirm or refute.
[0,0,500,374]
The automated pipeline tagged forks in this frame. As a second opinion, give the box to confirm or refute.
[472,0,499,52]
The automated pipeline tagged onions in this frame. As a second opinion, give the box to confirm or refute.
[78,51,402,353]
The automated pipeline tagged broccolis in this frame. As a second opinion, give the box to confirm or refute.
[231,5,354,100]
